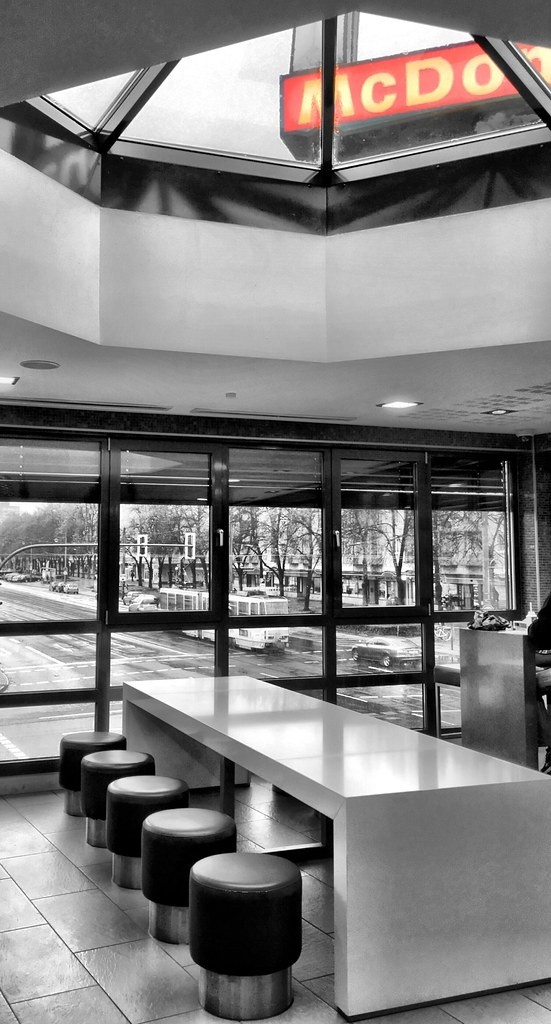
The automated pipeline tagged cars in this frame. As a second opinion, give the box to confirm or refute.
[49,581,79,594]
[5,573,41,583]
[123,590,160,613]
[351,636,422,670]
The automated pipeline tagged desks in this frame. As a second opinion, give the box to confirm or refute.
[121,676,551,1023]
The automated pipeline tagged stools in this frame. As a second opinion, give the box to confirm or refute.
[80,750,155,848]
[141,807,238,945]
[106,775,190,891]
[58,732,127,818]
[189,852,303,1021]
[271,783,334,850]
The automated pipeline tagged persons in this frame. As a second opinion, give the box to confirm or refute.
[526,592,551,774]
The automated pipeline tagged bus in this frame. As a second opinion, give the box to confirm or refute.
[158,587,290,654]
